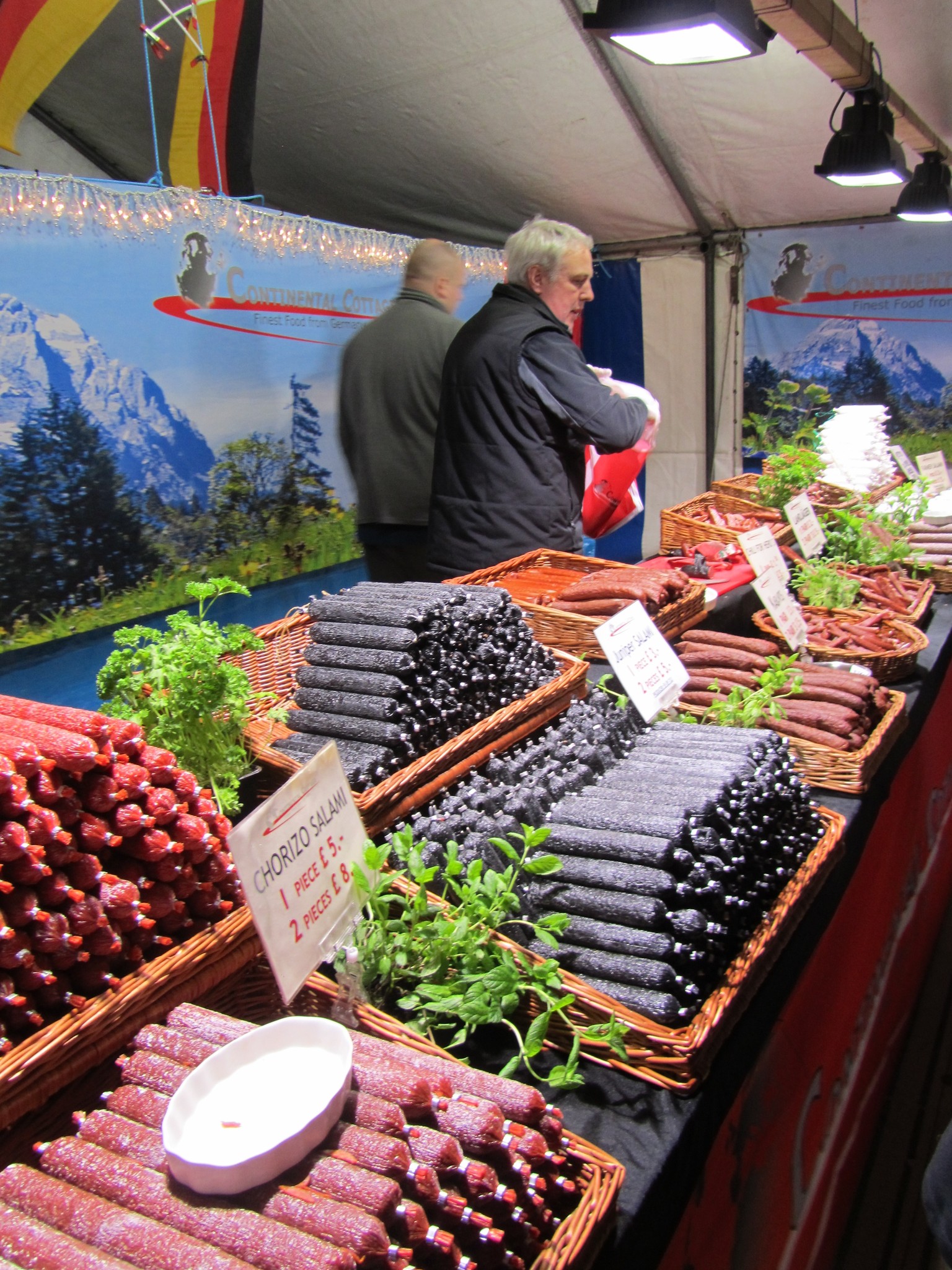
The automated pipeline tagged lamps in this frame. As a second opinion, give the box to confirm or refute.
[889,154,951,223]
[581,0,770,67]
[811,97,912,191]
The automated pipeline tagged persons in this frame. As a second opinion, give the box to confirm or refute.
[423,210,661,581]
[336,235,469,585]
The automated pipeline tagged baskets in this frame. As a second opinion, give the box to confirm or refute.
[0,446,952,1270]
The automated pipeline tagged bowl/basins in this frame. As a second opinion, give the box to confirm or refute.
[163,1015,352,1194]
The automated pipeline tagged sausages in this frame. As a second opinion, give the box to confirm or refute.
[0,447,947,1268]
